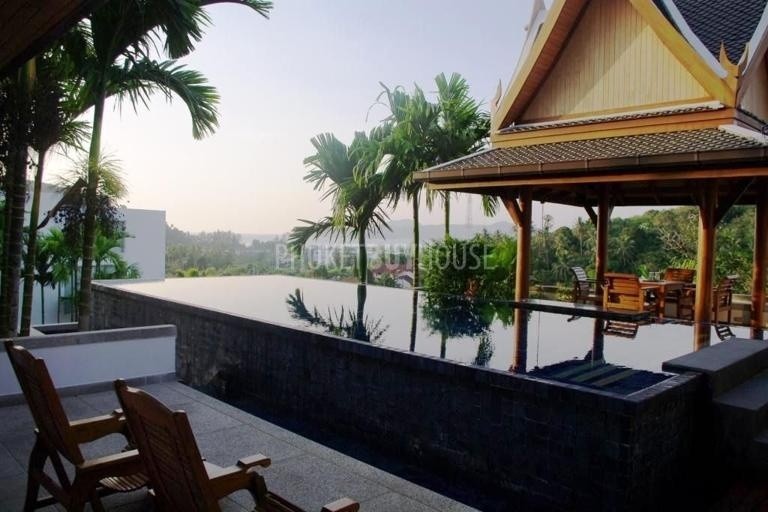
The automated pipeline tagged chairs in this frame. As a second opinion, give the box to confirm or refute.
[4,341,143,510]
[568,265,734,325]
[110,375,272,511]
[254,490,359,510]
